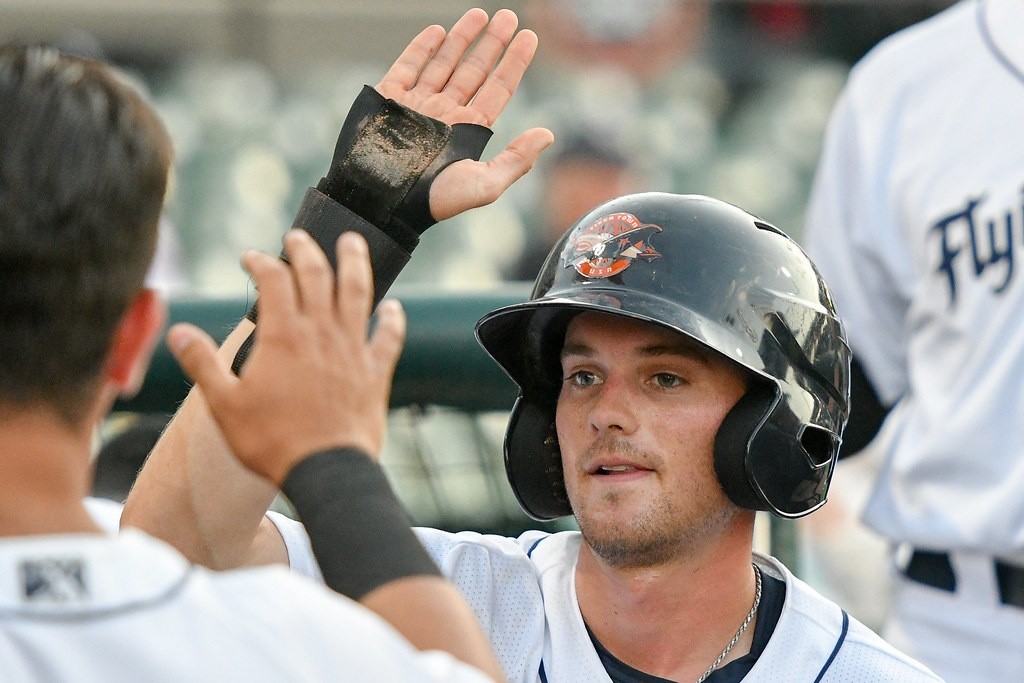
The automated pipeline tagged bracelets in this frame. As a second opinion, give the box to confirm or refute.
[280,444,444,604]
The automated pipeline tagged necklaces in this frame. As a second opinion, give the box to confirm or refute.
[695,564,762,683]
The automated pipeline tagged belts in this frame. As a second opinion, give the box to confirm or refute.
[904,550,1024,607]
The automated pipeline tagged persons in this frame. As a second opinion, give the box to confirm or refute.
[801,1,1024,683]
[116,5,943,683]
[1,42,511,683]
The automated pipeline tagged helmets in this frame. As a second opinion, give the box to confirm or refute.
[474,193,854,522]
